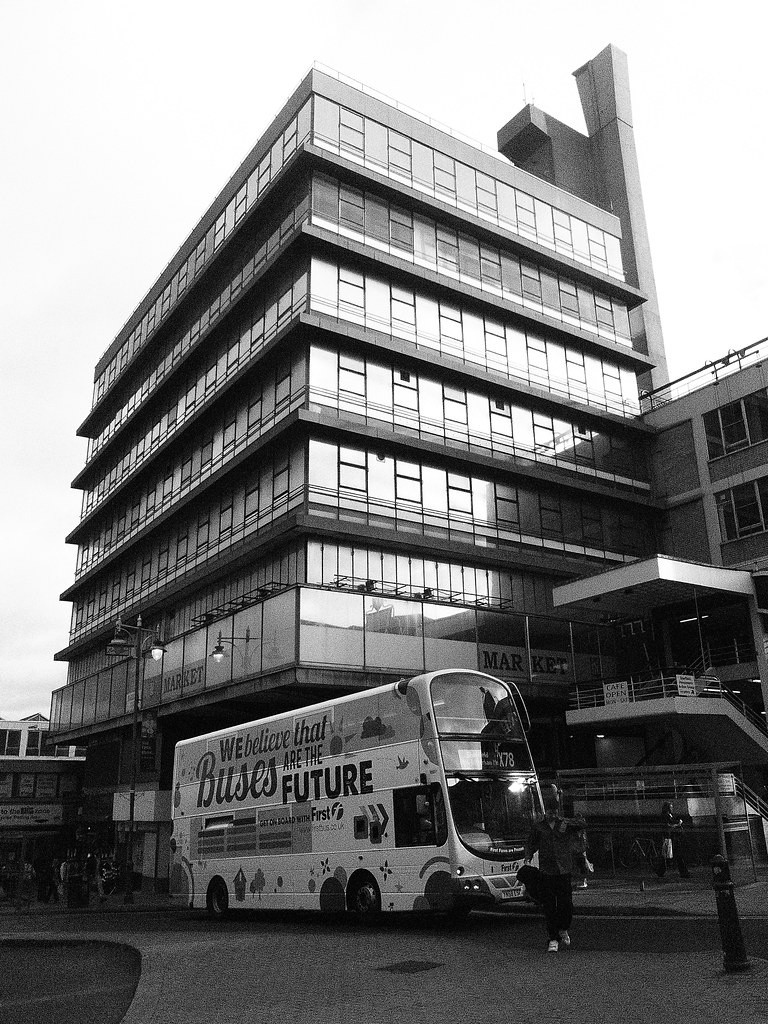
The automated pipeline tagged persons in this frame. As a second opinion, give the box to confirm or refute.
[653,801,693,878]
[0,852,117,905]
[525,799,594,954]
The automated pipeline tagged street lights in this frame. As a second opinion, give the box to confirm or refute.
[111,615,167,905]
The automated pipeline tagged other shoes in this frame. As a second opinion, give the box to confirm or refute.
[681,873,693,878]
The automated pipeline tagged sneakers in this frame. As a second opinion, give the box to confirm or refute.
[548,940,558,952]
[558,929,570,945]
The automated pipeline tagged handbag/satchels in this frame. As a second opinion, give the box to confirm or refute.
[662,838,673,859]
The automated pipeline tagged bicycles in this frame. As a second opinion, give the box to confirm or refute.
[618,830,673,872]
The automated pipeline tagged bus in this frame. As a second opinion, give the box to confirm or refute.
[170,668,564,928]
